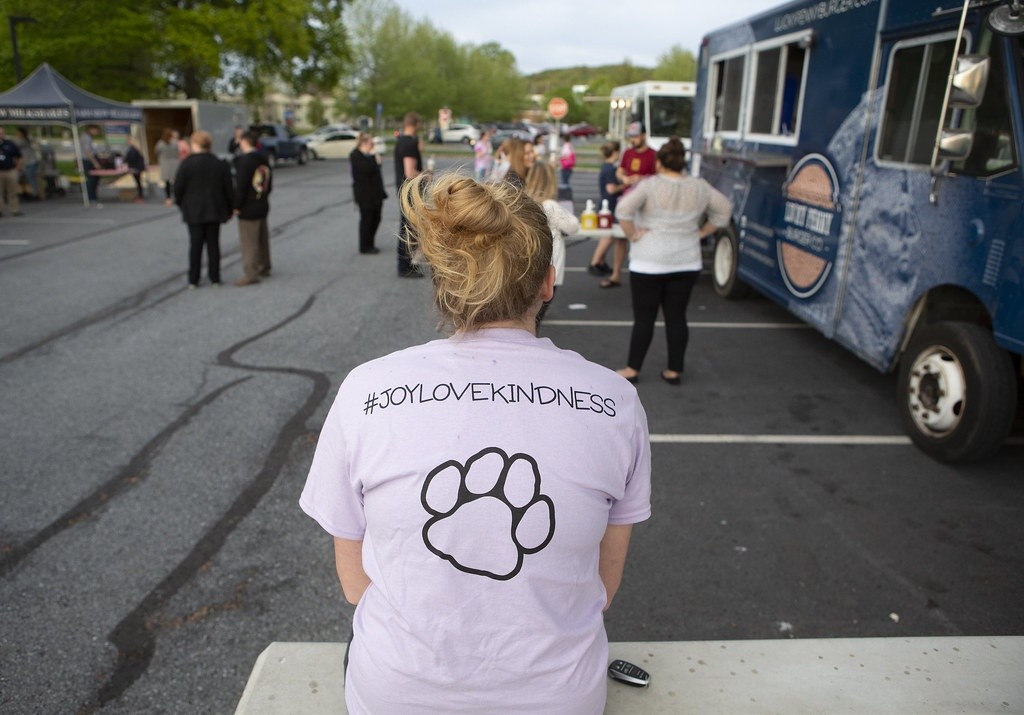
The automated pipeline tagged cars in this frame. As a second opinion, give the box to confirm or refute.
[251,124,308,169]
[305,130,387,159]
[424,119,597,144]
[295,123,354,143]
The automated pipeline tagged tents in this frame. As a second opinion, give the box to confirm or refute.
[0,62,149,207]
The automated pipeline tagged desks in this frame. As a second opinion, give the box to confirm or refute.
[564,223,627,249]
[89,168,131,177]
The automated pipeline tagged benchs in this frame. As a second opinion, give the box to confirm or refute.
[233,634,1023,714]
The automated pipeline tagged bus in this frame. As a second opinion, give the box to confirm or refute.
[689,0,1023,471]
[608,80,697,174]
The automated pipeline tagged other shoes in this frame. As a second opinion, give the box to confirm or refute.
[234,271,270,286]
[599,280,620,289]
[589,262,613,276]
[165,198,173,206]
[188,284,198,290]
[397,263,423,278]
[12,211,24,217]
[361,247,380,254]
[660,367,680,385]
[0,212,4,218]
[132,195,144,203]
[212,280,225,289]
[613,369,638,384]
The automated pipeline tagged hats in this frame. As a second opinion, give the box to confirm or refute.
[627,121,645,135]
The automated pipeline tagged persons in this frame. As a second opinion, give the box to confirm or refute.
[587,120,660,288]
[175,129,235,290]
[0,123,276,219]
[298,169,652,715]
[395,112,435,280]
[432,103,577,332]
[349,130,389,254]
[613,135,734,386]
[232,131,273,290]
